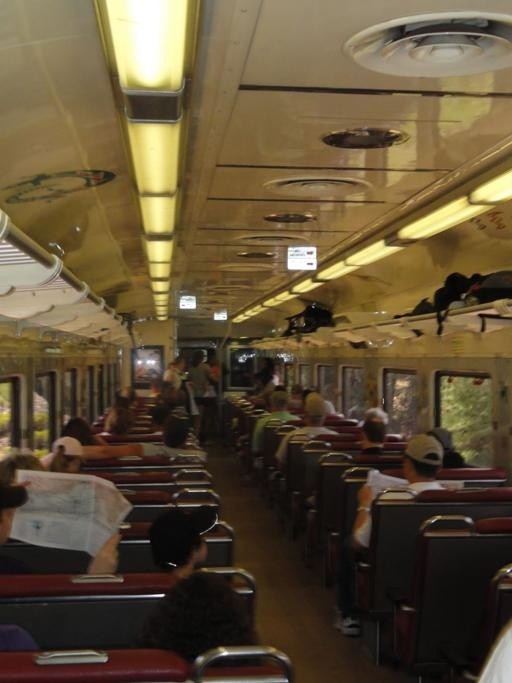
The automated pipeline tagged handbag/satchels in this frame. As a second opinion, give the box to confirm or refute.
[281,303,335,337]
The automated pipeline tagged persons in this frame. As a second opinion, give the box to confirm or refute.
[425,427,478,468]
[0,624,41,652]
[132,571,266,668]
[1,380,193,486]
[0,481,120,575]
[334,433,448,636]
[248,368,388,485]
[147,504,219,578]
[162,348,222,444]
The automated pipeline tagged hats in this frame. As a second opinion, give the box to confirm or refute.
[406,433,444,465]
[149,505,218,546]
[52,436,82,456]
[305,392,329,421]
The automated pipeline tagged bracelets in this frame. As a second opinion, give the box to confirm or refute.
[357,506,371,512]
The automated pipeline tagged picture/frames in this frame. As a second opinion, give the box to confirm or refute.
[131,345,164,390]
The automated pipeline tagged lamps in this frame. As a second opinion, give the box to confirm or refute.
[92,0,201,100]
[122,100,183,195]
[137,198,180,321]
[341,11,512,78]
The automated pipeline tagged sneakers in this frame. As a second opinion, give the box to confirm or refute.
[335,616,361,635]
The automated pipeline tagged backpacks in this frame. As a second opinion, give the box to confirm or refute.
[393,271,512,337]
